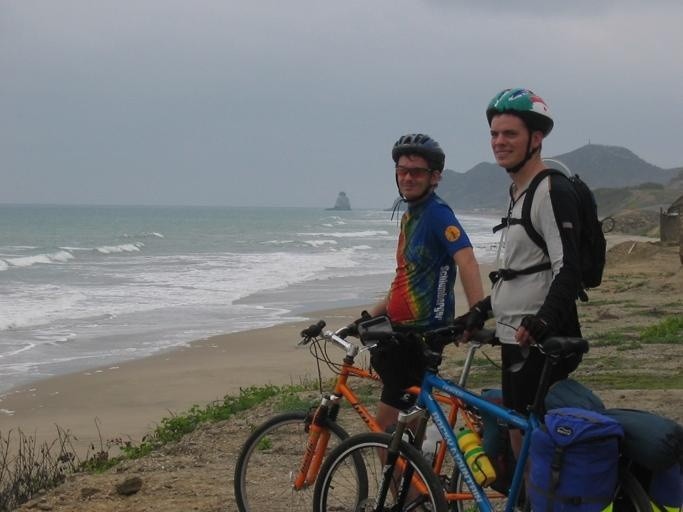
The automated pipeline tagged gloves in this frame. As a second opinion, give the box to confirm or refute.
[348,310,373,338]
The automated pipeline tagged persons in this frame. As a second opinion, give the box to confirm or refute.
[449,87,585,509]
[350,133,489,512]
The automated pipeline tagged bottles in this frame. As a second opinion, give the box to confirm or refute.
[456,426,497,489]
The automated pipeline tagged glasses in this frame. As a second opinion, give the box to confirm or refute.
[395,164,433,180]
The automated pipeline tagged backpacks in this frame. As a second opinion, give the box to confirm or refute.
[522,168,605,288]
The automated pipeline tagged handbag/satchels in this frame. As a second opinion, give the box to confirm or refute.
[529,406,624,511]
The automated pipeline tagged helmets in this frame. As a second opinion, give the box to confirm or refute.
[486,86,554,138]
[392,134,444,173]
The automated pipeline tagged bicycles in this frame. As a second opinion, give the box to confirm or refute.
[234,318,527,512]
[312,318,682,511]
